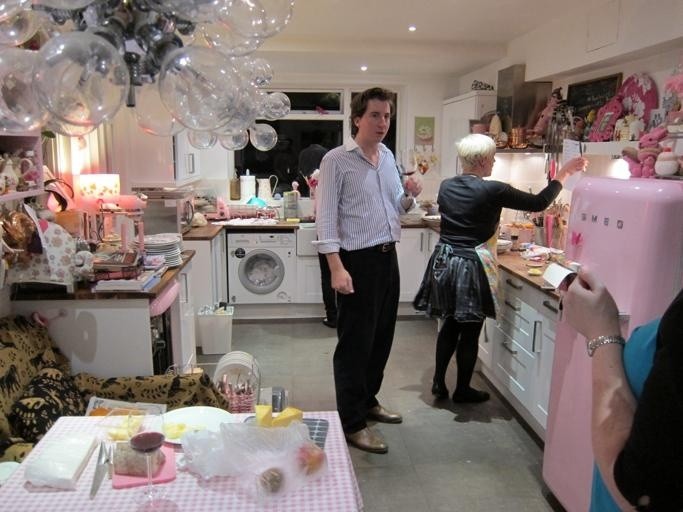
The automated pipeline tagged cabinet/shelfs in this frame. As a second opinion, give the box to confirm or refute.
[168,260,199,373]
[489,267,560,444]
[440,89,499,178]
[0,60,45,202]
[296,228,326,319]
[394,227,440,316]
[177,229,226,349]
[474,316,492,381]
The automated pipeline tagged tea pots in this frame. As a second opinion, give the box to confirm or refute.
[8,154,31,180]
[96,232,133,254]
[256,174,278,199]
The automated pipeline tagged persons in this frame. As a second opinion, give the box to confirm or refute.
[412,133,589,405]
[561,265,683,512]
[312,86,423,455]
[318,235,345,329]
[297,129,330,195]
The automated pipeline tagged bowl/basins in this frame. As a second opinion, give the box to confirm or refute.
[496,240,513,252]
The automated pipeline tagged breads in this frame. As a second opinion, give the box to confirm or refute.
[255,404,272,427]
[114,443,166,479]
[272,407,303,431]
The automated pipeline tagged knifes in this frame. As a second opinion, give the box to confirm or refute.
[91,440,109,499]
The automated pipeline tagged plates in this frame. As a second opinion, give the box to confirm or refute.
[212,351,260,380]
[130,234,183,266]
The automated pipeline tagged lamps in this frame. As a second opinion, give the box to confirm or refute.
[0,0,295,151]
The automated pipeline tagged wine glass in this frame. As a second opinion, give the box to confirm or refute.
[122,403,178,511]
[396,148,420,209]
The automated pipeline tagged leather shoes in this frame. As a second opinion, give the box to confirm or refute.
[344,426,388,454]
[323,317,336,328]
[432,384,449,401]
[363,404,402,424]
[452,390,490,404]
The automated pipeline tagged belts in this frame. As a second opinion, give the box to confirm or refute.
[371,242,396,252]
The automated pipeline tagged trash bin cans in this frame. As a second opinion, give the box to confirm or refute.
[198,305,234,355]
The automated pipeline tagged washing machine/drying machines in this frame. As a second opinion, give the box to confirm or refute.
[226,233,296,318]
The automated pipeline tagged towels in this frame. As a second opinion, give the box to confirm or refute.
[24,430,98,489]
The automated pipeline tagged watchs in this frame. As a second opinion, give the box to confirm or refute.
[587,335,624,357]
